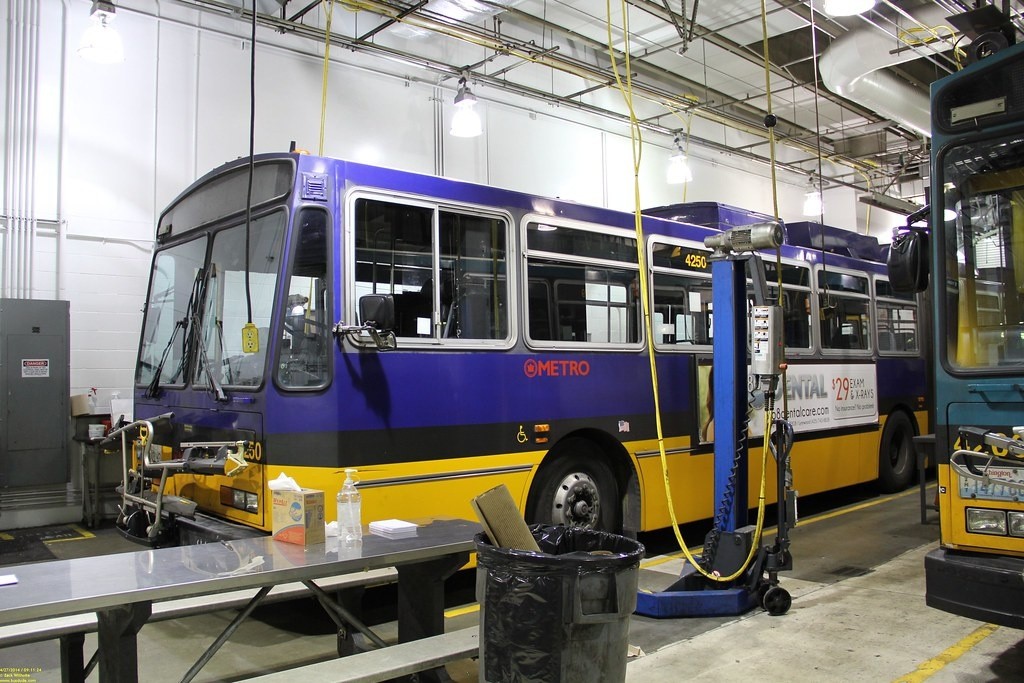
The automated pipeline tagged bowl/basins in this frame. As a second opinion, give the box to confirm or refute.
[88,424,105,437]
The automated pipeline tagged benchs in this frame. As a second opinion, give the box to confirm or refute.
[235,621,481,683]
[0,565,399,683]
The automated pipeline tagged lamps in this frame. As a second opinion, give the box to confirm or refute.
[669,129,688,163]
[803,175,823,197]
[453,72,478,106]
[88,0,116,29]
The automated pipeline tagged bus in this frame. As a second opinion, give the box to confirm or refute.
[919,41,1024,627]
[115,153,921,635]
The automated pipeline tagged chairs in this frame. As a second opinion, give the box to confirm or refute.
[350,277,921,365]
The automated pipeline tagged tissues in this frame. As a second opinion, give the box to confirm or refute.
[267,472,326,547]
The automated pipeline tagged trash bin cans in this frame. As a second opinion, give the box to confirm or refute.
[474,523,646,682]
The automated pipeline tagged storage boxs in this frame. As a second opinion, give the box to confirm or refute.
[273,488,325,545]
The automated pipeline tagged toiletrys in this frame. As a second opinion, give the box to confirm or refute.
[335,467,362,541]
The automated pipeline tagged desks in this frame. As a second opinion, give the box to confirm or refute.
[1,517,488,683]
